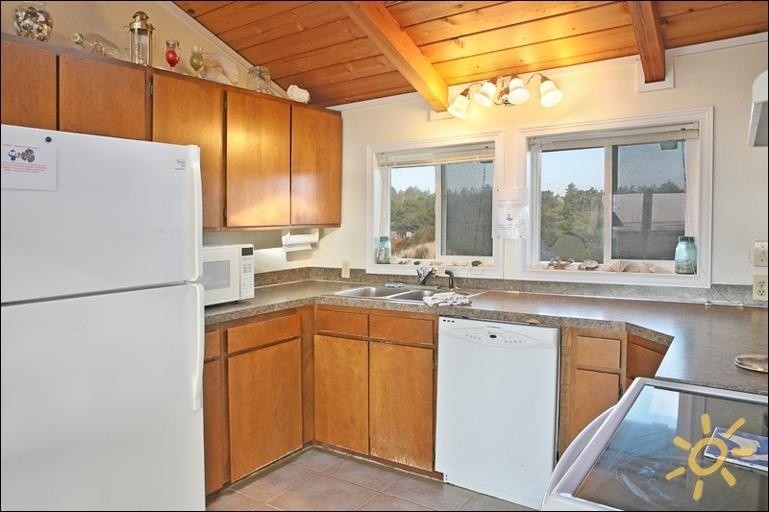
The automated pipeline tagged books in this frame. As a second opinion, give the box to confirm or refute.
[704,426,768,472]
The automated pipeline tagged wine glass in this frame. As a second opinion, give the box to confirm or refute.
[165,39,181,71]
[190,45,204,79]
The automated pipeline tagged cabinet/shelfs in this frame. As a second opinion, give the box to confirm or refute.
[1,39,344,233]
[560,327,668,463]
[308,306,433,497]
[206,304,314,499]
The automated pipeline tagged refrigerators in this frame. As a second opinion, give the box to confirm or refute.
[1,124,207,512]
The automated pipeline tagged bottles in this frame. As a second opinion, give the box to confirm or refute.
[71,31,122,61]
[675,236,697,275]
[376,236,391,264]
[12,0,54,42]
[248,65,271,95]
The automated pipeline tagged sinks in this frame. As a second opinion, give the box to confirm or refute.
[321,283,409,299]
[389,288,439,305]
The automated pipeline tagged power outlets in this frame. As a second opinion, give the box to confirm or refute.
[754,275,768,302]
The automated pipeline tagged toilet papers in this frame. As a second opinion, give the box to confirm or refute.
[281,233,318,253]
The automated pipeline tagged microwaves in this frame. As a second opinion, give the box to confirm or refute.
[201,243,255,306]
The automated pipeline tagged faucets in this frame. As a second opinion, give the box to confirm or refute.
[416,266,436,286]
[445,270,454,290]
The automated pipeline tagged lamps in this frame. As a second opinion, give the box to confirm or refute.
[446,72,563,120]
